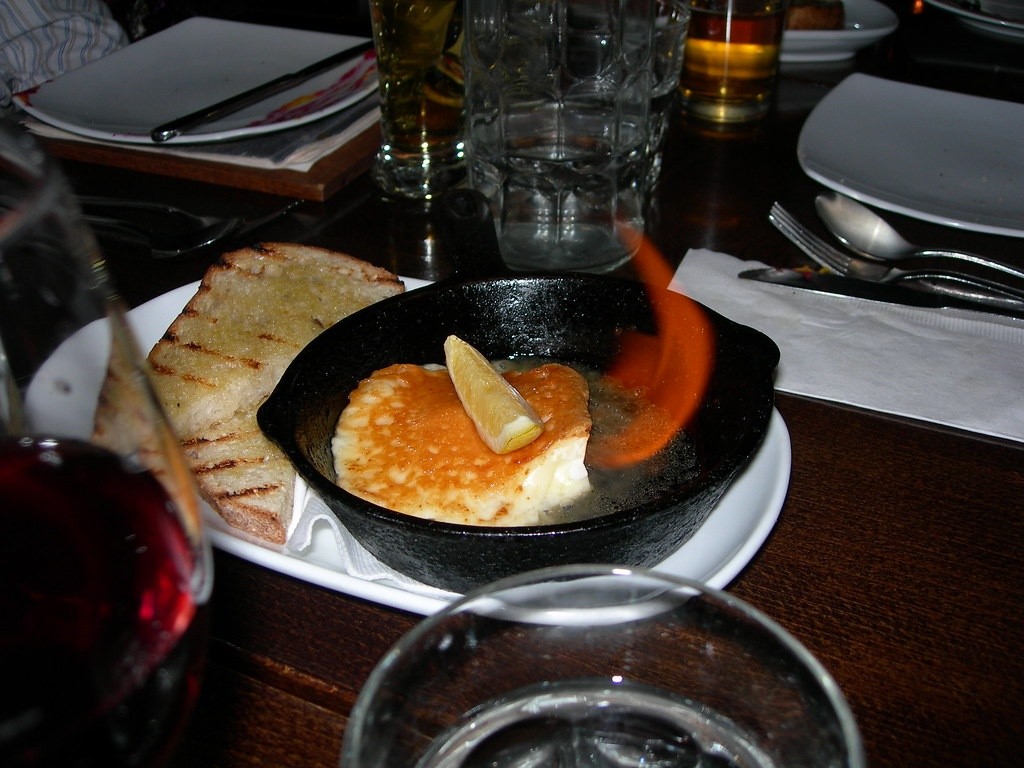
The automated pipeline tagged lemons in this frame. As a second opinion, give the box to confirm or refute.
[443,333,543,453]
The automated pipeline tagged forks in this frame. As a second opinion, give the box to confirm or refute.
[766,199,1024,309]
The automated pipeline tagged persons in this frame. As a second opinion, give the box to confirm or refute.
[0,0,132,130]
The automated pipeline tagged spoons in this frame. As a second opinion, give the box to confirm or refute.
[813,189,1024,283]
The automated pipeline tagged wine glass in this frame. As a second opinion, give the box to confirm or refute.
[2,141,218,768]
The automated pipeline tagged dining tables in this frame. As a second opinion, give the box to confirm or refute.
[2,0,1023,767]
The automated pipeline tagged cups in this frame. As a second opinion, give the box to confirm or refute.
[370,0,793,275]
[339,563,863,767]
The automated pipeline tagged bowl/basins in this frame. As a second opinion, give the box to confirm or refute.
[257,275,783,595]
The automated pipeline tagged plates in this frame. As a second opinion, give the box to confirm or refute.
[27,278,797,628]
[926,0,1022,38]
[796,71,1024,240]
[778,0,899,61]
[12,14,377,144]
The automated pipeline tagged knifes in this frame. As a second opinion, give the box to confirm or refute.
[146,34,379,141]
[736,268,1024,324]
